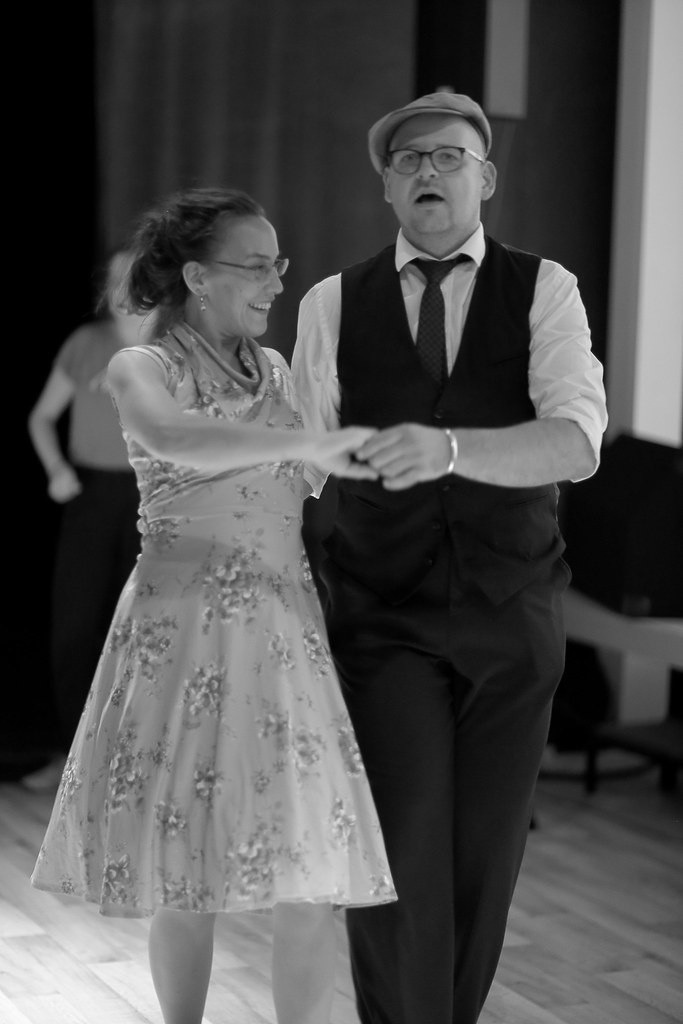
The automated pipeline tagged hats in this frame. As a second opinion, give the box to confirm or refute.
[368,92,492,177]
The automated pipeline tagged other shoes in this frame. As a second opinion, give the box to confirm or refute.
[24,755,68,789]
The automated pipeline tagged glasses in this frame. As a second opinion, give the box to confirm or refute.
[214,257,289,277]
[388,147,485,174]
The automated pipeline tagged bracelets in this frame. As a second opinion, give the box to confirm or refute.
[445,429,457,474]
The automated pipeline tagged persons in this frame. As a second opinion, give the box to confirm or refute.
[291,90,609,1024]
[30,186,398,1023]
[24,240,164,791]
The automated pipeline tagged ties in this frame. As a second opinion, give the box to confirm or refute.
[409,254,473,385]
